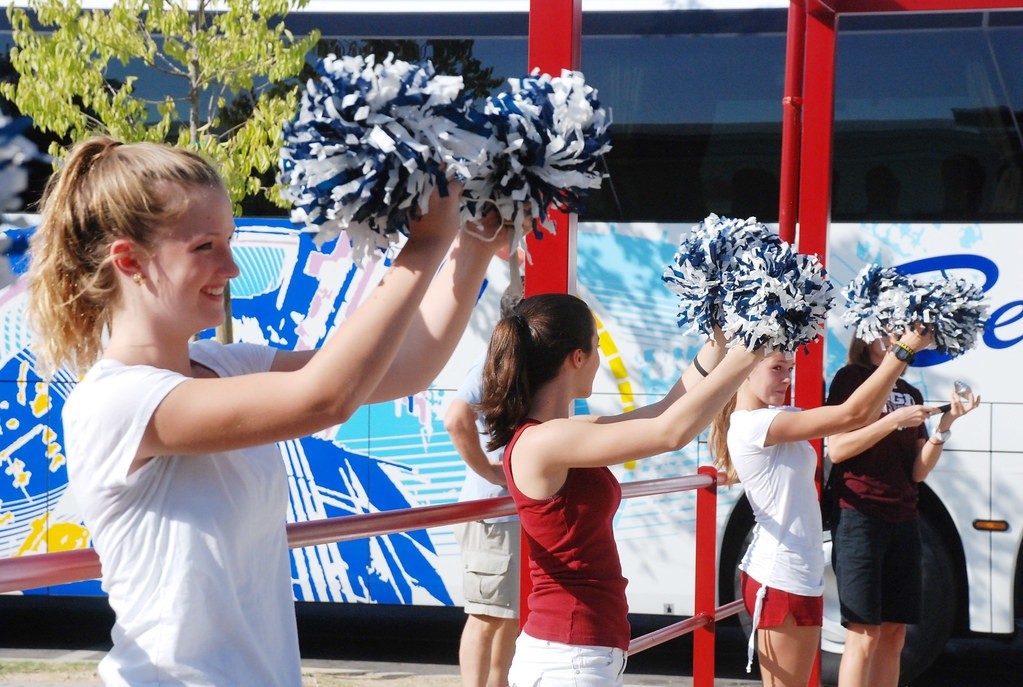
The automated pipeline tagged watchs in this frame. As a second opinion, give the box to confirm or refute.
[932,427,952,441]
[889,344,915,365]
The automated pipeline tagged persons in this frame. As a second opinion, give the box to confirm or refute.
[31,135,533,686]
[442,268,982,687]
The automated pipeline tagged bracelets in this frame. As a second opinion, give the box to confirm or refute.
[927,438,945,446]
[896,340,915,357]
[694,356,711,382]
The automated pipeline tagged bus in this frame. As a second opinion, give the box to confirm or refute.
[1,0,1023,686]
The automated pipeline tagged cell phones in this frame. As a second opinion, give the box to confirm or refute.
[929,404,952,415]
